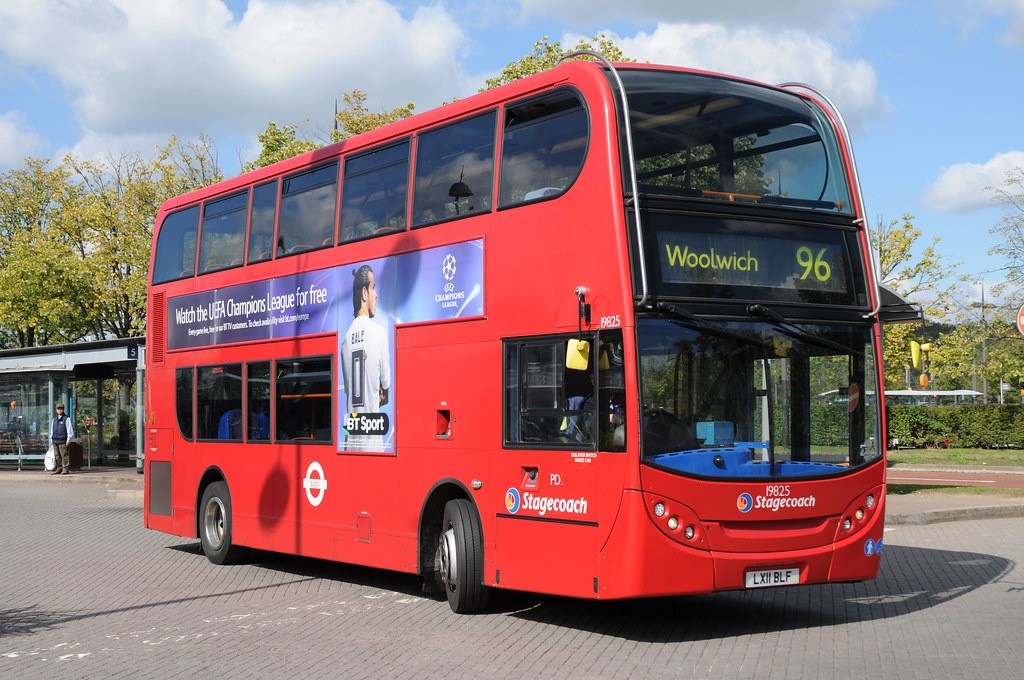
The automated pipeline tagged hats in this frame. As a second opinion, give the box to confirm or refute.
[56,403,64,408]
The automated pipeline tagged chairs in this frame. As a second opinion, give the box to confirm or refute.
[217,409,270,440]
[177,226,401,278]
[522,185,562,201]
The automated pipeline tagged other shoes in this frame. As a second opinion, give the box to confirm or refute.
[61,468,69,474]
[51,468,62,475]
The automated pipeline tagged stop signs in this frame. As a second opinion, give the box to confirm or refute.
[847,385,860,411]
[1017,303,1024,337]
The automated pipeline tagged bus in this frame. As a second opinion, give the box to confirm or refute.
[144,51,931,613]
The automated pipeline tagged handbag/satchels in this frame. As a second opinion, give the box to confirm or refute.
[45,445,56,471]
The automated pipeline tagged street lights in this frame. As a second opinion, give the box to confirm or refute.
[977,281,988,405]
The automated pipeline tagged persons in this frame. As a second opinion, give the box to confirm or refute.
[50,403,74,474]
[559,368,623,444]
[341,265,391,451]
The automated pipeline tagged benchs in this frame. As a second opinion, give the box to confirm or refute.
[0,432,49,455]
